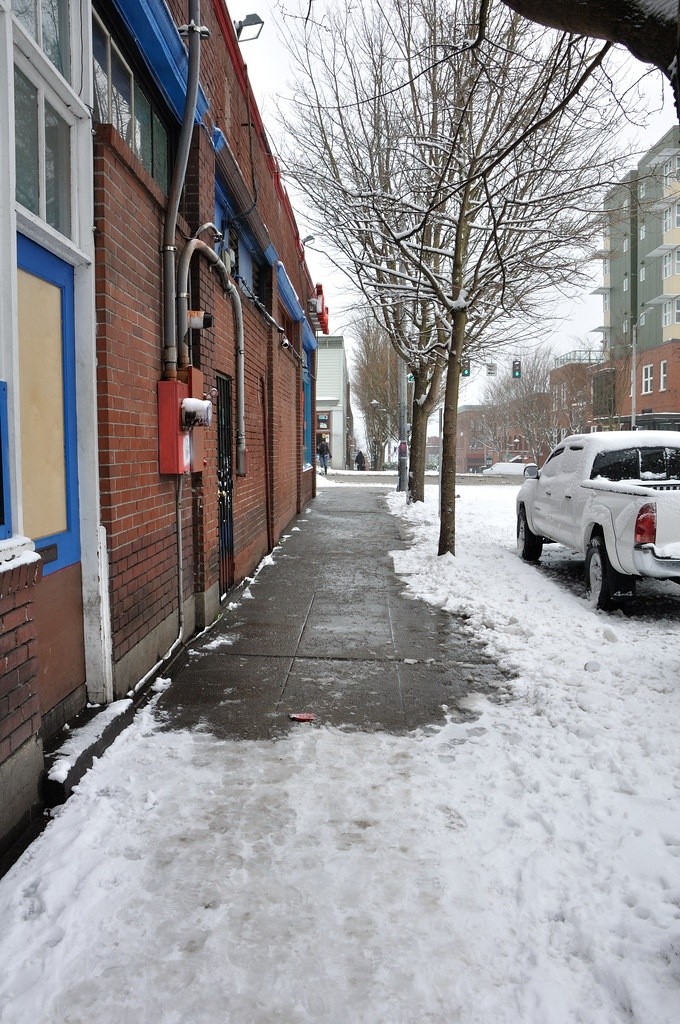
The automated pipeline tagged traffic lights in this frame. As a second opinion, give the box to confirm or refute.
[461,357,470,376]
[513,361,521,379]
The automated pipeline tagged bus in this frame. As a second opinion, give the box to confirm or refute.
[583,412,680,431]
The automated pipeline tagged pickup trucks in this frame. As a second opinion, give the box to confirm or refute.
[516,431,680,611]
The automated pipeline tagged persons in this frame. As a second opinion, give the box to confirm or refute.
[354,451,366,471]
[317,438,332,475]
